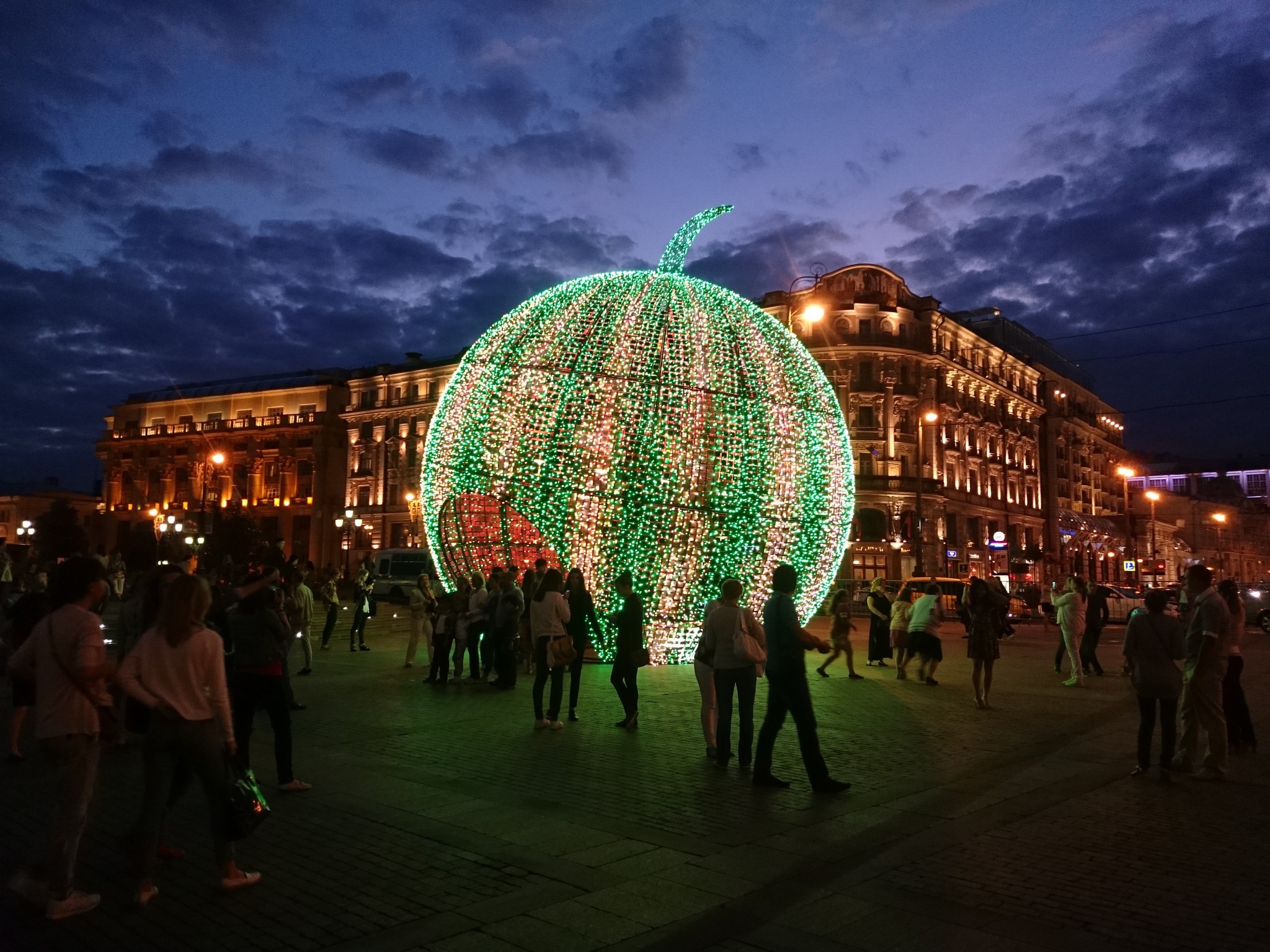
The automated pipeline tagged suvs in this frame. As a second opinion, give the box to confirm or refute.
[897,578,1032,621]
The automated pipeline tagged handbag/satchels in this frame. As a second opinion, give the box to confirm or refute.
[628,648,651,669]
[365,600,377,617]
[322,601,331,612]
[221,747,271,839]
[732,606,766,664]
[546,635,578,669]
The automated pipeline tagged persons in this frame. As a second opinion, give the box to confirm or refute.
[0,496,1260,919]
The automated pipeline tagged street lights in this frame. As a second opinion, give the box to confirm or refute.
[193,441,223,571]
[1117,467,1134,587]
[1146,492,1159,585]
[335,508,363,588]
[1213,515,1225,581]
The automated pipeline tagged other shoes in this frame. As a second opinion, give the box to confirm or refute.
[297,667,312,675]
[359,643,371,651]
[739,764,750,769]
[812,776,852,795]
[350,643,357,651]
[220,871,262,890]
[422,674,517,691]
[706,747,735,757]
[1129,751,1227,781]
[45,889,101,919]
[550,720,564,729]
[534,718,551,728]
[278,778,312,790]
[289,701,304,710]
[616,710,639,730]
[135,885,159,907]
[816,660,942,686]
[321,645,334,651]
[752,773,791,788]
[716,761,726,769]
[1052,665,1131,687]
[545,709,552,718]
[569,711,579,721]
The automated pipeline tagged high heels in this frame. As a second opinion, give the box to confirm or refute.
[974,698,992,710]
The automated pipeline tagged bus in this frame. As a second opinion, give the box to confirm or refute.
[372,549,457,609]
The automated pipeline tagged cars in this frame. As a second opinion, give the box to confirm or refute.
[853,585,898,606]
[1242,589,1270,633]
[1050,584,1179,625]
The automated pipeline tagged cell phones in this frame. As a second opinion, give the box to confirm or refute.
[1052,581,1056,590]
